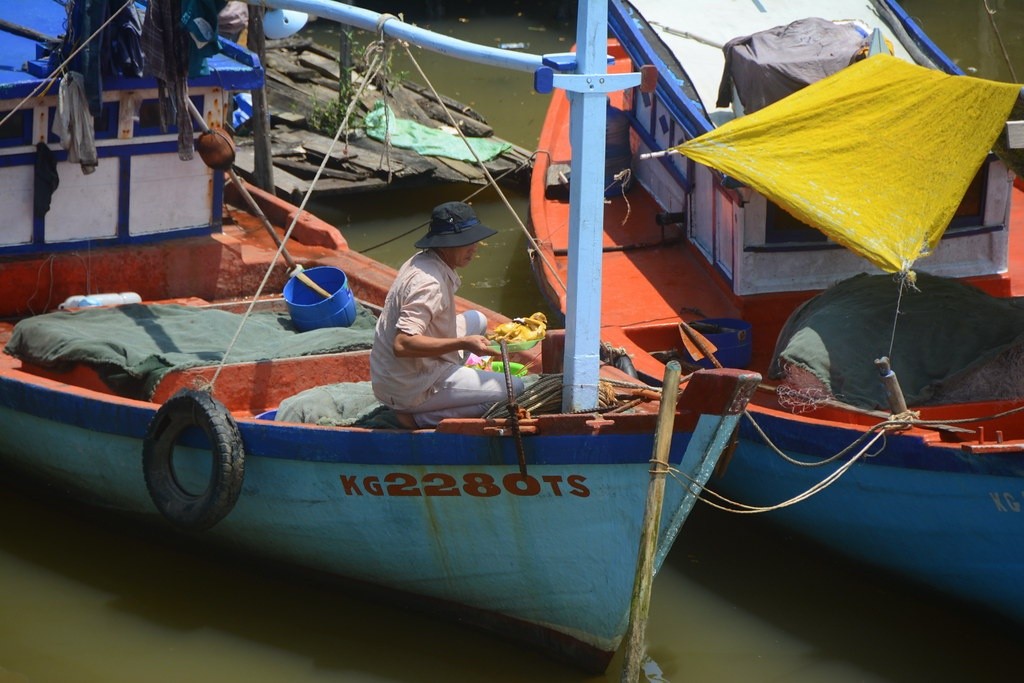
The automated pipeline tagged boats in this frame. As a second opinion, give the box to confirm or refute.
[522,0,1024,622]
[0,0,767,675]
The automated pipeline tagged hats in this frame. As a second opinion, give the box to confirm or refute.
[415,202,498,248]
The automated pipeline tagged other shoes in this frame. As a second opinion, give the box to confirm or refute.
[394,414,420,430]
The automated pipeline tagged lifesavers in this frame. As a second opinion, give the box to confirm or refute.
[141,388,248,533]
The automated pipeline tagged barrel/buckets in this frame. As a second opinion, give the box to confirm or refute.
[283,266,356,331]
[683,317,753,368]
[606,97,635,197]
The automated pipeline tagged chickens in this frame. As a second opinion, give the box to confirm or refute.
[486,312,547,345]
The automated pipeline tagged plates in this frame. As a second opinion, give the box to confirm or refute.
[484,339,541,354]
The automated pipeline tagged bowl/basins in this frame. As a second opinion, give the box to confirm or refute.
[470,360,528,377]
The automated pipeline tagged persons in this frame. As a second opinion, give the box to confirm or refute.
[371,201,525,430]
